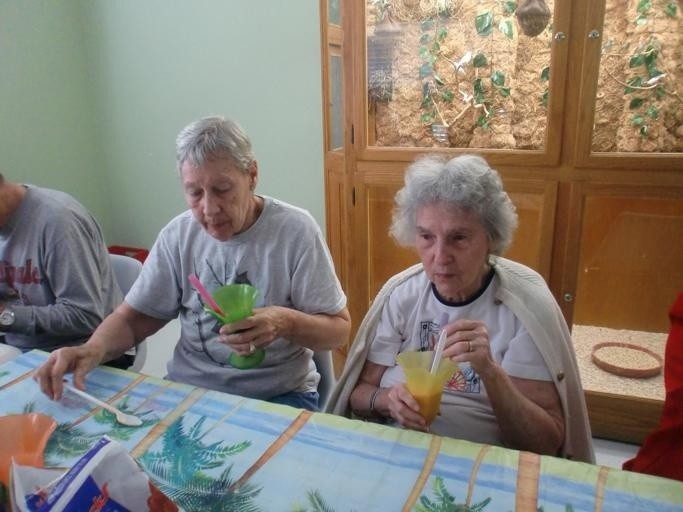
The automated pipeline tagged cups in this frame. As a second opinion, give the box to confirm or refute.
[0,412,57,488]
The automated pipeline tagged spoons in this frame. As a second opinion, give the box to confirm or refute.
[62,383,144,427]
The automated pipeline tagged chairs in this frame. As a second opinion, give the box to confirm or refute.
[104,253,149,372]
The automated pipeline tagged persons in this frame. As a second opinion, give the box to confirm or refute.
[31,116,352,412]
[0,175,138,373]
[323,153,597,463]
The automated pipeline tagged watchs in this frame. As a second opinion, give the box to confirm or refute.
[0,302,16,332]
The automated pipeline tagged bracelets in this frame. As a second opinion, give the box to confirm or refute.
[370,384,382,419]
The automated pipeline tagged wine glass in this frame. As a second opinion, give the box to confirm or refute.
[203,283,266,369]
[396,350,454,434]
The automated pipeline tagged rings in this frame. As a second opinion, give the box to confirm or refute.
[248,341,257,354]
[469,340,474,354]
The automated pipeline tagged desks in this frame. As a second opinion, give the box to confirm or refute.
[1,349,682,512]
